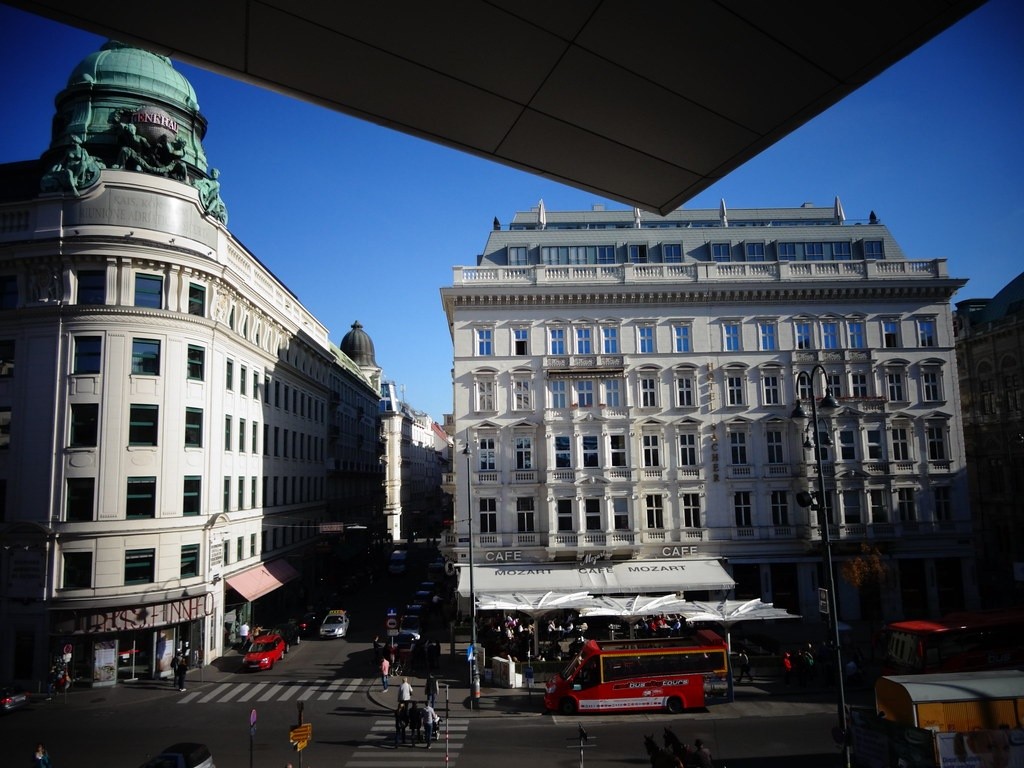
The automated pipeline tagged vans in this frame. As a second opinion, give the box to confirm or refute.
[388,550,408,574]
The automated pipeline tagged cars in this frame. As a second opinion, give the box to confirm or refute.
[268,623,301,653]
[320,610,351,639]
[294,612,323,639]
[0,686,32,713]
[399,615,422,640]
[404,555,446,616]
[242,634,285,671]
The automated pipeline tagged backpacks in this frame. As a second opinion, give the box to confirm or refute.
[396,717,406,729]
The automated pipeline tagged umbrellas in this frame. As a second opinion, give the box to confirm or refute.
[680,597,801,653]
[475,590,594,657]
[577,593,705,639]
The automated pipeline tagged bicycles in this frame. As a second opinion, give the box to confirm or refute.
[389,660,403,677]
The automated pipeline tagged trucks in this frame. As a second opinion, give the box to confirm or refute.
[140,742,216,768]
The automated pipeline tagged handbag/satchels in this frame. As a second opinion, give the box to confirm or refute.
[410,721,416,729]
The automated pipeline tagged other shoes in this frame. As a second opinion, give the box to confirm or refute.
[387,689,388,691]
[383,690,387,693]
[181,689,186,692]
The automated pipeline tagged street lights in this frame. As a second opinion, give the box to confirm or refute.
[789,363,857,768]
[462,428,480,709]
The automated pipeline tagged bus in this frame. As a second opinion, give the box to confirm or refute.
[875,606,1024,682]
[547,629,734,715]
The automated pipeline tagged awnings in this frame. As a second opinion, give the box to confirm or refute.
[226,557,298,602]
[457,560,737,598]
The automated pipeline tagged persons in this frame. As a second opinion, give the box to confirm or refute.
[250,623,263,641]
[171,651,183,687]
[178,658,187,692]
[44,666,57,700]
[736,639,837,692]
[239,621,250,650]
[34,744,49,768]
[372,634,441,751]
[477,613,684,660]
[691,739,713,768]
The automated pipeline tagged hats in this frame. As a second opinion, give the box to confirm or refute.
[402,678,407,681]
